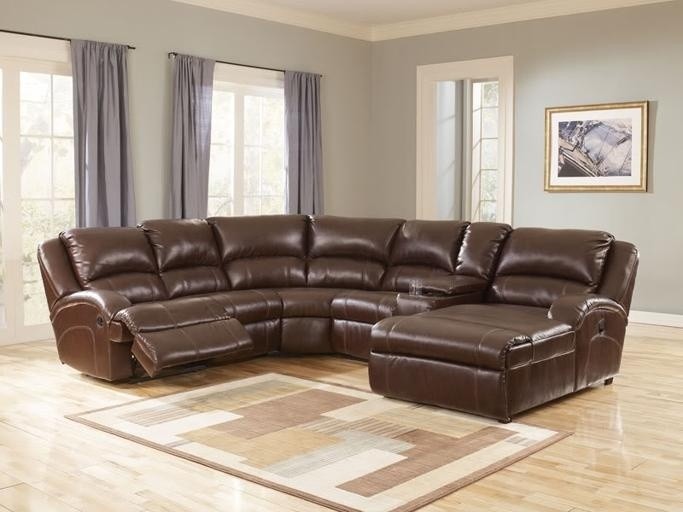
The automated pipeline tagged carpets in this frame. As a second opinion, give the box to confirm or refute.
[64,370,575,512]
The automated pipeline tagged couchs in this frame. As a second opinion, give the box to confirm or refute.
[38,213,640,423]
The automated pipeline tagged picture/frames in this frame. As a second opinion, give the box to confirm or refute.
[544,101,649,193]
